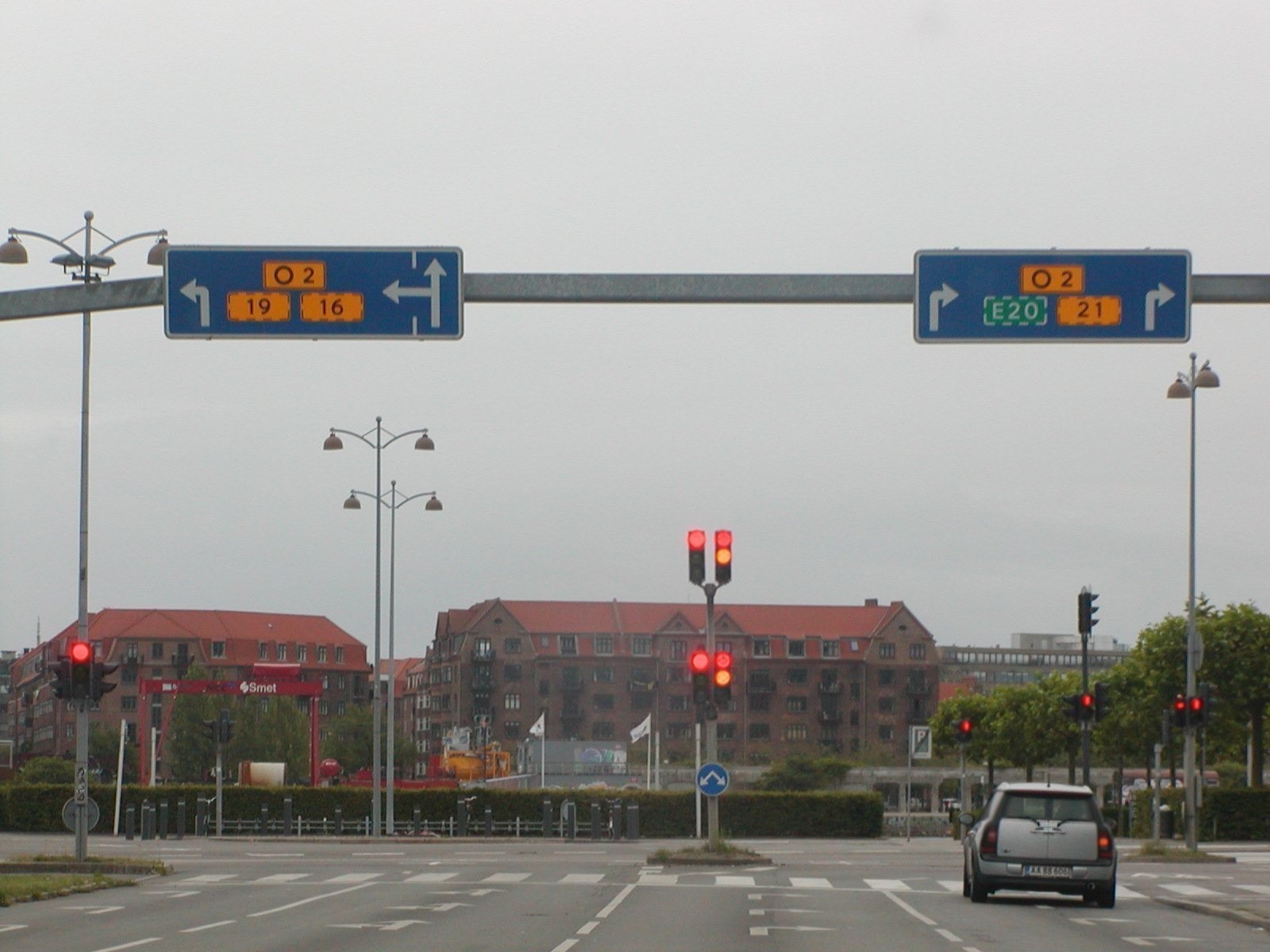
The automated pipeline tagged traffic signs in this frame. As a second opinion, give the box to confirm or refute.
[163,247,463,340]
[913,250,1191,344]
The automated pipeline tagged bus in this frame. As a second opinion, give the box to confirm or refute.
[435,744,510,781]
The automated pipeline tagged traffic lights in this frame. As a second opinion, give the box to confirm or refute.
[202,717,237,743]
[949,716,975,742]
[691,649,733,705]
[685,529,731,586]
[46,642,121,702]
[1061,694,1112,725]
[1173,678,1221,728]
[1078,591,1102,633]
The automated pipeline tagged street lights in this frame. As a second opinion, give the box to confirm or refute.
[1,211,172,868]
[342,480,445,838]
[321,415,436,838]
[1163,350,1222,852]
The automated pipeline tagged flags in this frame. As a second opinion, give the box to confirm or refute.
[630,715,652,744]
[529,713,545,736]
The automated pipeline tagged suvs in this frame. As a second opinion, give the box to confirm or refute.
[960,781,1120,908]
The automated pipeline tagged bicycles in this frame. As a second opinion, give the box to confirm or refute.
[197,795,217,836]
[461,794,477,836]
[603,796,622,838]
